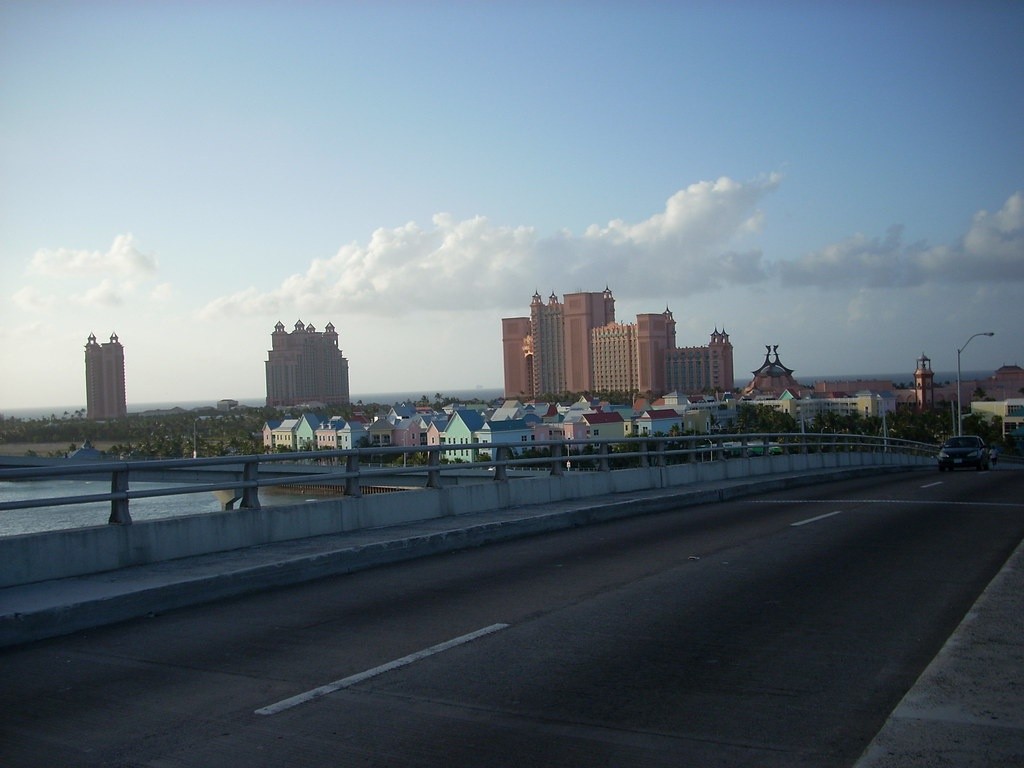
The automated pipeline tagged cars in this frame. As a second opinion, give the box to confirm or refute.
[937,435,991,472]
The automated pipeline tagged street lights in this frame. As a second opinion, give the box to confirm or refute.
[957,331,994,436]
[193,418,201,458]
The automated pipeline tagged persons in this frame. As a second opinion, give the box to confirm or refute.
[989,445,999,468]
